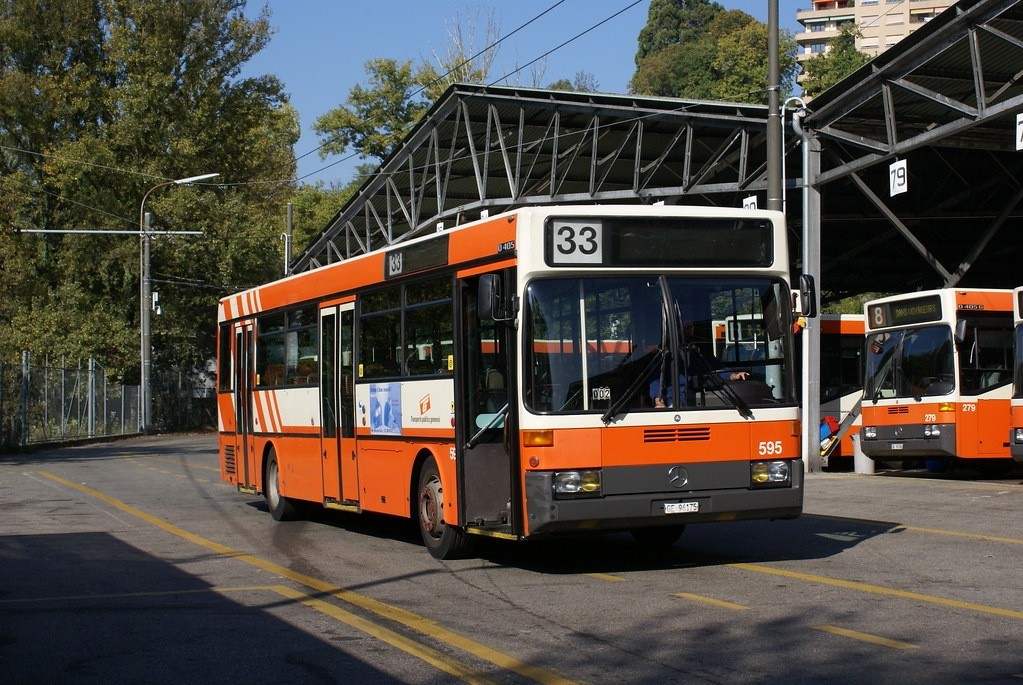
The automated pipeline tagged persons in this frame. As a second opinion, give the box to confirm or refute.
[650,359,750,408]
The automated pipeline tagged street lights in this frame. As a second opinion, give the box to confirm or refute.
[142,172,221,428]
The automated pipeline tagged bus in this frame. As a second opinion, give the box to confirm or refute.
[719,313,869,475]
[215,196,818,561]
[711,320,726,363]
[860,287,1023,476]
[342,338,632,392]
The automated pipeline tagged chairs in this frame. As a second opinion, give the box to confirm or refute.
[265,362,353,402]
